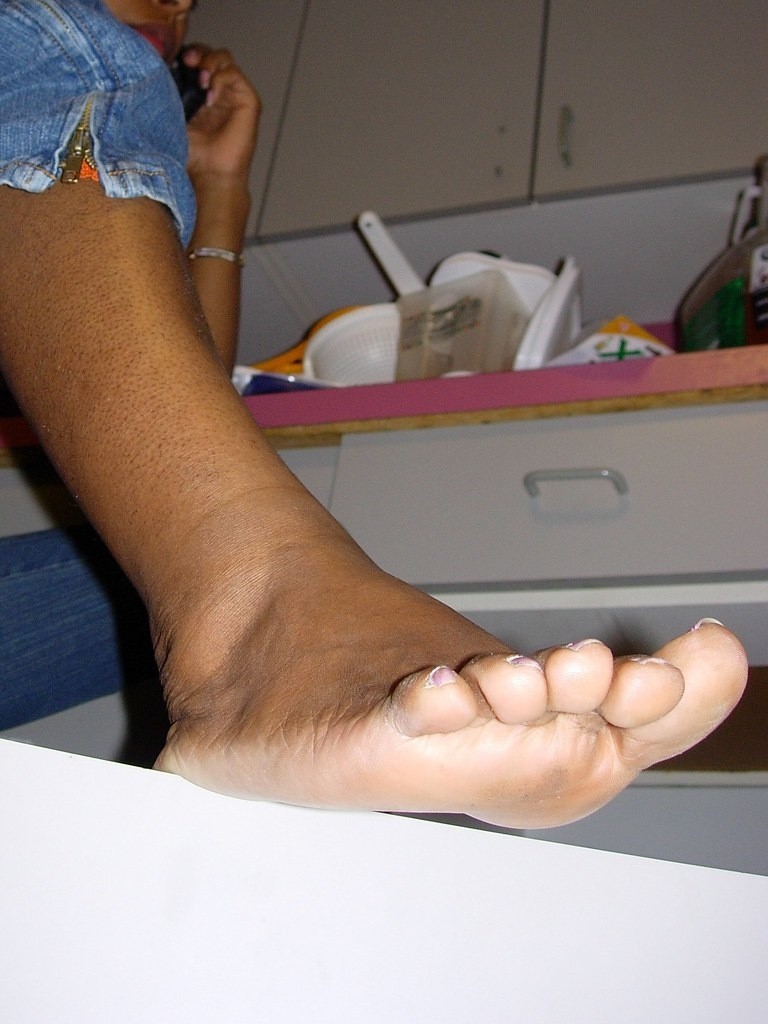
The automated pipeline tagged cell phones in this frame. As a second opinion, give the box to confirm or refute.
[169,48,207,122]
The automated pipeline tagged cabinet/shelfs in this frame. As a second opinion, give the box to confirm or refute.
[243,344,768,876]
[181,0,768,366]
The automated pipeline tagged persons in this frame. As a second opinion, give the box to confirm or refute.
[0,0,748,827]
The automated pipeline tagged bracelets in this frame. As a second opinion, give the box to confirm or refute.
[188,246,246,266]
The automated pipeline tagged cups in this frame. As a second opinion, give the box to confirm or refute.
[394,269,533,379]
